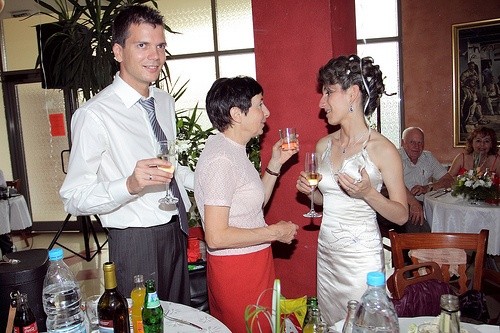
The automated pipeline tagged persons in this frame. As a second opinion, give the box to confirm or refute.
[194,76,300,333]
[60,5,194,307]
[0,169,13,256]
[447,126,500,178]
[391,126,460,283]
[296,54,408,328]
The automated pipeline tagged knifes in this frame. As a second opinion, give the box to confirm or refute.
[434,190,451,198]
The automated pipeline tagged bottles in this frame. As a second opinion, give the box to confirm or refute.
[302,294,318,329]
[42,247,87,333]
[141,279,164,333]
[484,168,493,188]
[130,275,147,333]
[302,308,323,333]
[351,271,399,333]
[341,300,359,333]
[97,262,130,333]
[440,294,461,333]
[12,293,39,333]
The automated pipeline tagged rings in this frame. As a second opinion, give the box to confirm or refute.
[149,175,152,181]
[345,187,350,192]
[353,180,357,184]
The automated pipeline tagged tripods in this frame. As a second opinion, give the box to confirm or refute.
[48,89,109,261]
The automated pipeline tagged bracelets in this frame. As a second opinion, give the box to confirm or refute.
[265,168,281,178]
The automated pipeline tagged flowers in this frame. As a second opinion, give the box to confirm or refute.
[453,151,497,202]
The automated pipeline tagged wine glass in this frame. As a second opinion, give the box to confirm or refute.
[156,141,179,205]
[302,152,323,218]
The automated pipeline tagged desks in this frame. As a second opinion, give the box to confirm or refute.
[82,293,232,333]
[421,188,500,274]
[0,194,32,235]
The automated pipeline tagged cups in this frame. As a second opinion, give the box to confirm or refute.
[84,294,102,333]
[417,323,440,333]
[279,127,297,151]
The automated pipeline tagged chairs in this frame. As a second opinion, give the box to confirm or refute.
[388,226,490,297]
[6,178,30,247]
[387,261,484,324]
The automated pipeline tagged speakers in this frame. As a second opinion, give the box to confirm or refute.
[36,20,90,90]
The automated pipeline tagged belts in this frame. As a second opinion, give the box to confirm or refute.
[170,214,180,222]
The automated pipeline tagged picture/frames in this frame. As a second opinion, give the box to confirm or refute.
[451,17,500,152]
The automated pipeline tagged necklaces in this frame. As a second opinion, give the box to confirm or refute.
[339,127,367,153]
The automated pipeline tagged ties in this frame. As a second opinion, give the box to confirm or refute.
[139,97,190,237]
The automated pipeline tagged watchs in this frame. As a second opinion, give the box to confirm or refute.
[426,184,434,192]
